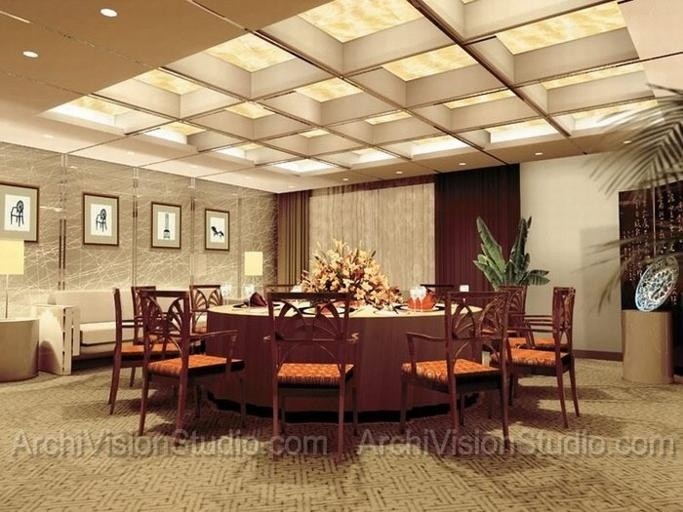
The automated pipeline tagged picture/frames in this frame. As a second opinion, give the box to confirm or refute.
[151,202,181,249]
[205,208,230,251]
[83,193,119,246]
[0,182,39,243]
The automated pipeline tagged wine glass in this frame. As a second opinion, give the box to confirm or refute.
[293,285,305,310]
[244,284,255,311]
[419,286,427,313]
[410,286,420,315]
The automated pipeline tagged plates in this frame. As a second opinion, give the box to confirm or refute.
[635,254,679,312]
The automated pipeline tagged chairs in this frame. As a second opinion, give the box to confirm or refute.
[399,290,511,455]
[189,285,223,334]
[108,288,181,418]
[132,286,204,389]
[502,287,568,393]
[265,284,297,302]
[484,287,580,429]
[262,293,360,459]
[420,284,453,304]
[138,288,244,445]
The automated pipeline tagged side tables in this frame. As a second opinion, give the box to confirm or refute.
[0,319,39,382]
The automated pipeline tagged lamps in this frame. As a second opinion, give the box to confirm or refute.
[244,251,262,294]
[0,241,24,318]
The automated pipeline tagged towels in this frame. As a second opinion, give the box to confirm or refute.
[244,292,267,307]
[428,289,440,308]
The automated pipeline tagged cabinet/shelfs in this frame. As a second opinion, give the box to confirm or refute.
[622,310,674,384]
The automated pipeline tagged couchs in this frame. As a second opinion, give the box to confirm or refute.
[32,288,249,376]
